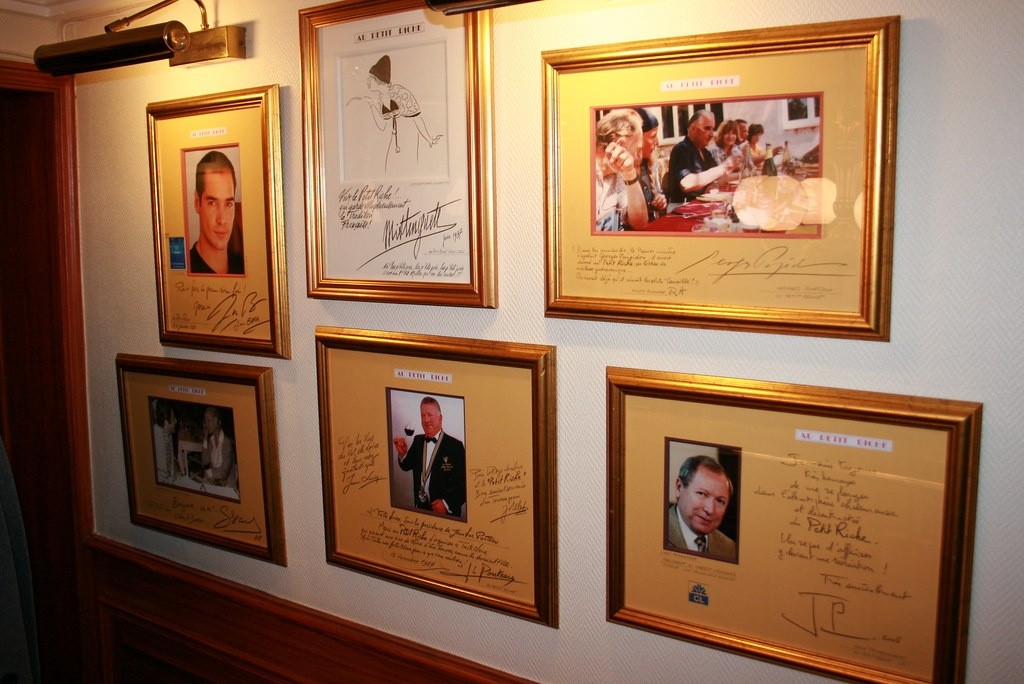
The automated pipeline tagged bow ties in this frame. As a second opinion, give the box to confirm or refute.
[425,436,437,444]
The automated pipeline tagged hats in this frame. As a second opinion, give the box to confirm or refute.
[635,107,658,132]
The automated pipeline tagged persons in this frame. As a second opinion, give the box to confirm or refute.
[203,407,232,486]
[748,122,783,168]
[634,106,669,222]
[735,119,756,176]
[709,118,756,187]
[393,397,466,518]
[153,398,177,483]
[596,109,649,231]
[0,430,44,684]
[190,151,244,275]
[668,455,737,563]
[669,109,744,203]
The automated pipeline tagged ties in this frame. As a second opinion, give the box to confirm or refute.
[694,535,707,553]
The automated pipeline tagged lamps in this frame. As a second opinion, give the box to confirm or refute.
[35,0,247,81]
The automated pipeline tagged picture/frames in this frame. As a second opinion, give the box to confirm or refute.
[312,325,564,629]
[606,365,985,682]
[116,354,288,568]
[541,15,902,342]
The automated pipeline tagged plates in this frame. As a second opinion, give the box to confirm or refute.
[696,194,729,201]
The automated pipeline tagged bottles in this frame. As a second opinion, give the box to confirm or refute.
[739,145,756,180]
[782,141,790,171]
[762,143,777,176]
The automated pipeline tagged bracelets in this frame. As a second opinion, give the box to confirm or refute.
[624,175,638,185]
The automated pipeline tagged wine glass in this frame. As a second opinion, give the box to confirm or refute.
[404,422,415,440]
[708,181,719,208]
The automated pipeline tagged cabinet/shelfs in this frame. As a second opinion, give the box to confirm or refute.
[299,2,499,310]
[146,84,291,363]
